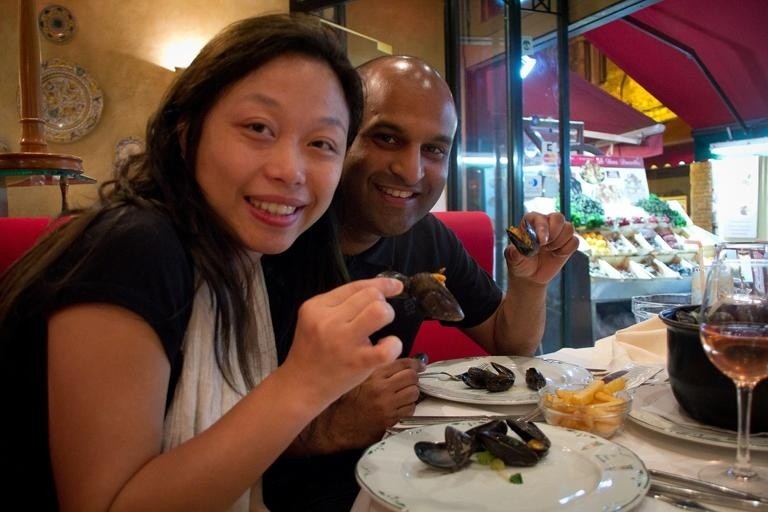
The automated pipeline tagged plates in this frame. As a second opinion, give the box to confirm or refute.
[416,354,593,406]
[626,382,768,452]
[353,417,651,512]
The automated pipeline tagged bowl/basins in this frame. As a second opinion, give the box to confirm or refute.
[631,292,692,324]
[538,382,633,438]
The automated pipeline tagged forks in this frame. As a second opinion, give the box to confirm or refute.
[401,405,543,423]
[415,363,491,381]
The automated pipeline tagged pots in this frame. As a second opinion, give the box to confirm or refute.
[657,303,768,434]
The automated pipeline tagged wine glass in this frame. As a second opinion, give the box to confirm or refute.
[698,258,768,494]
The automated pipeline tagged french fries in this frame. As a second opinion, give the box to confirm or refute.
[541,379,631,435]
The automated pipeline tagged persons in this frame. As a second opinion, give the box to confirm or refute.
[0,12,404,511]
[261,53,580,511]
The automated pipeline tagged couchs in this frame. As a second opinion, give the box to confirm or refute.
[1,211,495,365]
